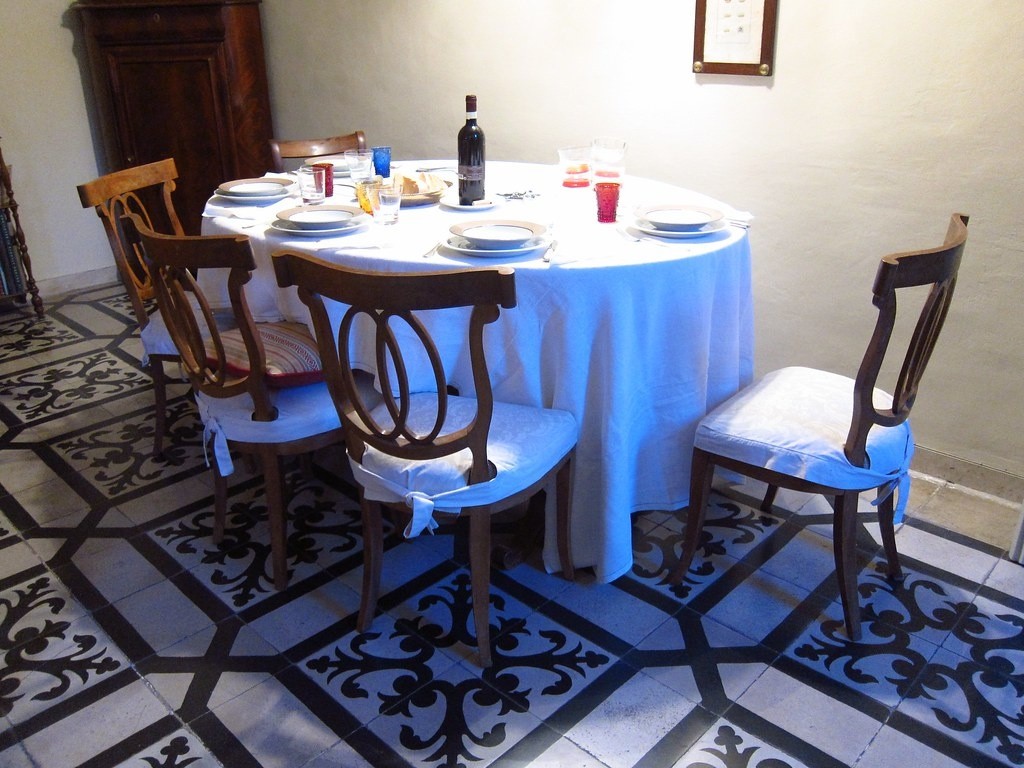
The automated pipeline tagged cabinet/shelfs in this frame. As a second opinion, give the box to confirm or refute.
[63,0,276,288]
[691,0,779,77]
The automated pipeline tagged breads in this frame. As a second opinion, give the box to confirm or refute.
[391,170,449,195]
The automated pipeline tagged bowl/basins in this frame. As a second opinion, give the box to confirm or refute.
[275,204,362,230]
[304,154,360,171]
[450,219,546,250]
[218,178,293,196]
[643,205,724,232]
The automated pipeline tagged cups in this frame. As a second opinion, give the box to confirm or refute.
[367,181,402,226]
[345,145,392,216]
[594,182,620,224]
[295,160,334,205]
[555,136,626,189]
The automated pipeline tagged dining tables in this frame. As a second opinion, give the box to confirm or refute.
[197,160,755,584]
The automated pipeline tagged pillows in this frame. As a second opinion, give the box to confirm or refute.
[199,319,361,387]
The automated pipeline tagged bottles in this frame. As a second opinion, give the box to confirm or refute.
[457,94,486,206]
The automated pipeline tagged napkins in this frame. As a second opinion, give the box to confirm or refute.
[726,210,754,228]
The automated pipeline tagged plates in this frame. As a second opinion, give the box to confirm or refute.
[442,234,553,259]
[630,215,730,239]
[299,164,351,178]
[270,218,366,236]
[213,187,291,205]
[439,195,500,211]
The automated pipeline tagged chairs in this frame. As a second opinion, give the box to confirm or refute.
[269,130,369,173]
[121,215,410,583]
[665,213,969,642]
[272,247,582,667]
[78,157,234,459]
